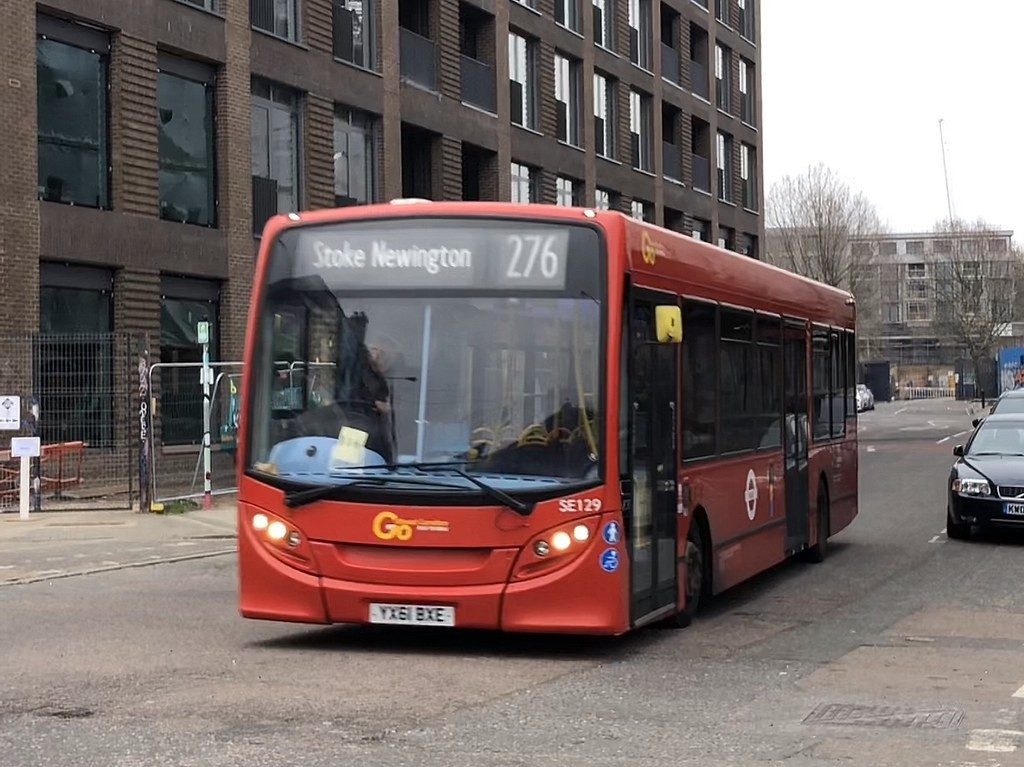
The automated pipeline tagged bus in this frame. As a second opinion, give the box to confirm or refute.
[219,198,859,638]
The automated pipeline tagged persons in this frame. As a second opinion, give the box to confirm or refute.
[317,325,417,454]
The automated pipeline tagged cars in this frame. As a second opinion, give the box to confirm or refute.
[857,384,875,411]
[947,413,1024,540]
[972,390,1024,430]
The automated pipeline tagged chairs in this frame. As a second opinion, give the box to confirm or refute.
[464,420,593,478]
[996,429,1021,442]
